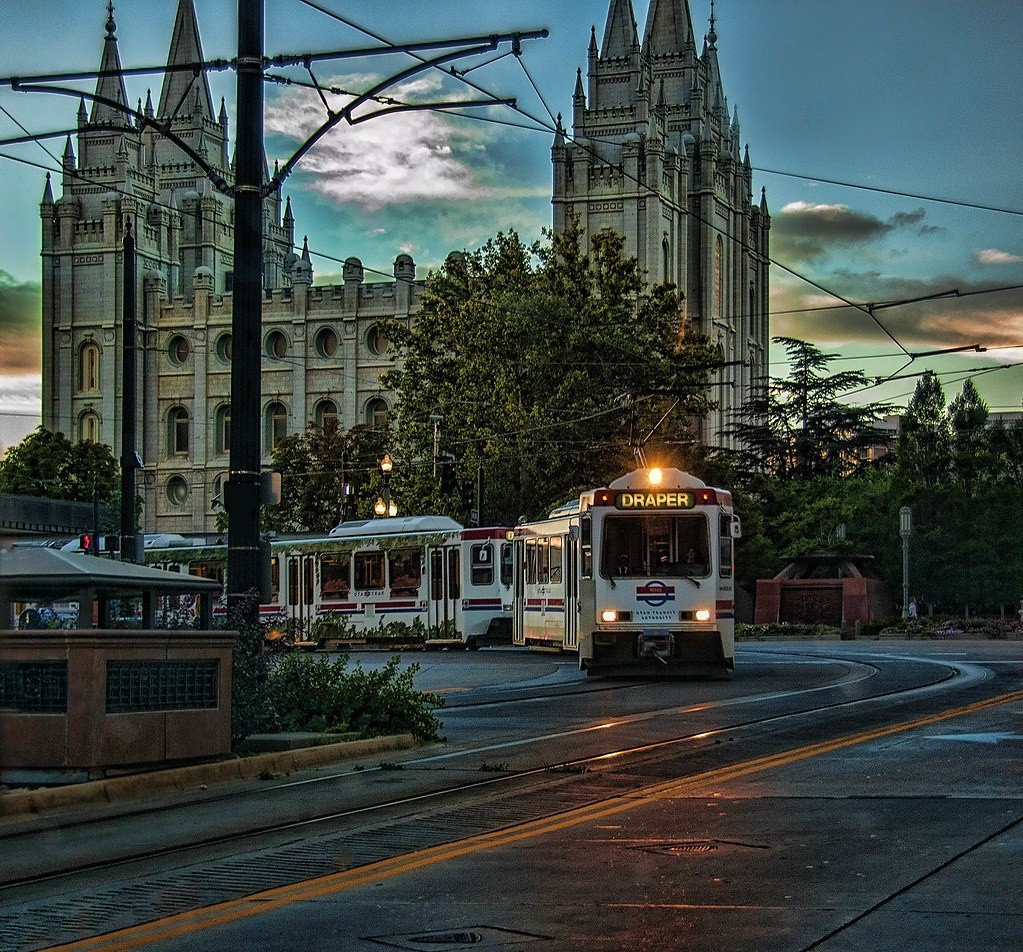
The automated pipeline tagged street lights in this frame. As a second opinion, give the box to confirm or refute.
[900,507,912,620]
[375,454,399,520]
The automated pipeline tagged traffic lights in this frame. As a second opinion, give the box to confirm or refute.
[79,533,93,549]
[462,477,473,510]
[442,455,456,489]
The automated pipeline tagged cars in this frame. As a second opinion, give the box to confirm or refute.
[36,606,78,622]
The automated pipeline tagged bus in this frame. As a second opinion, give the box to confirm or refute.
[11,464,741,683]
